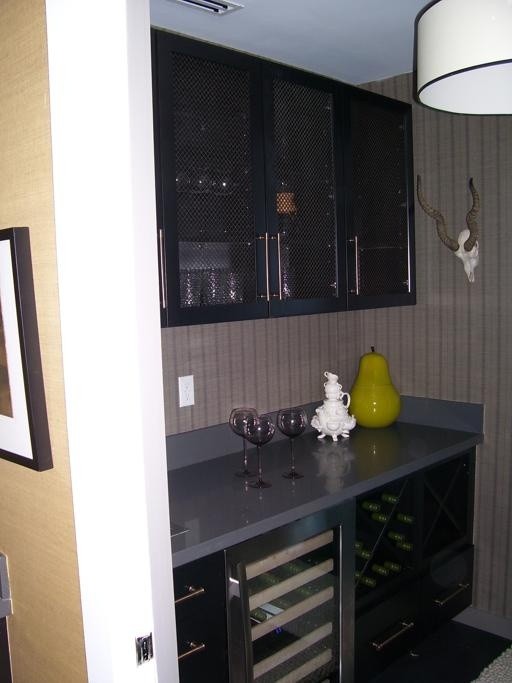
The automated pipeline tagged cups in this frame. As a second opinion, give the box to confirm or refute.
[181,266,250,306]
[176,162,249,196]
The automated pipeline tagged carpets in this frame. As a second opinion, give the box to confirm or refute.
[472,644,512,683]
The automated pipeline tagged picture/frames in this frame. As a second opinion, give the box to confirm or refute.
[0,225,53,471]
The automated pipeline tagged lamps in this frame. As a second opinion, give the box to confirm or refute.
[414,0,511,114]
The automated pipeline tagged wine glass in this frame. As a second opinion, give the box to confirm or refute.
[229,406,308,488]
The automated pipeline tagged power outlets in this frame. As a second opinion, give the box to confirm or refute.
[177,374,196,408]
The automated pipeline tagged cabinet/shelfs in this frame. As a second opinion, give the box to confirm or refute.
[326,79,416,312]
[150,26,349,329]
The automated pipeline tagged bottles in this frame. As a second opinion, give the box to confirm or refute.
[356,492,415,590]
[246,561,315,622]
